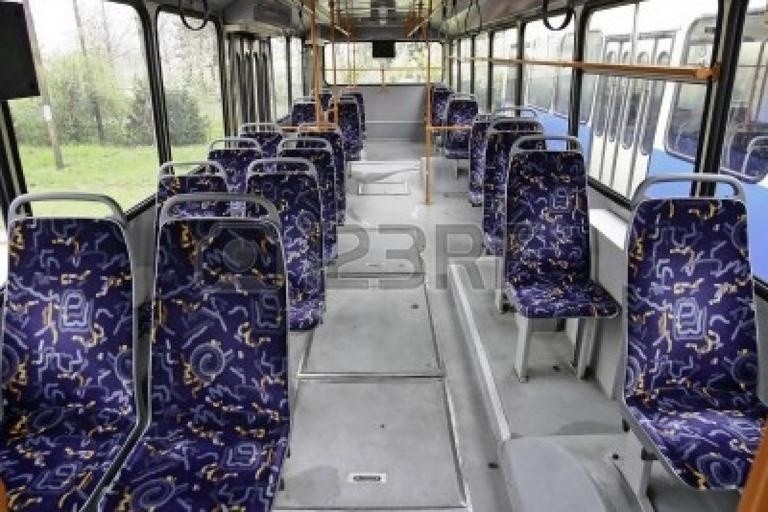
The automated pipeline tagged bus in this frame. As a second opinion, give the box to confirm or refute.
[499,0,768,283]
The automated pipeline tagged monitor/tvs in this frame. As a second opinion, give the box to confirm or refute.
[374,40,395,58]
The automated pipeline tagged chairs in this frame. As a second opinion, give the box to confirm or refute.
[93,189,297,512]
[427,83,768,511]
[0,189,145,511]
